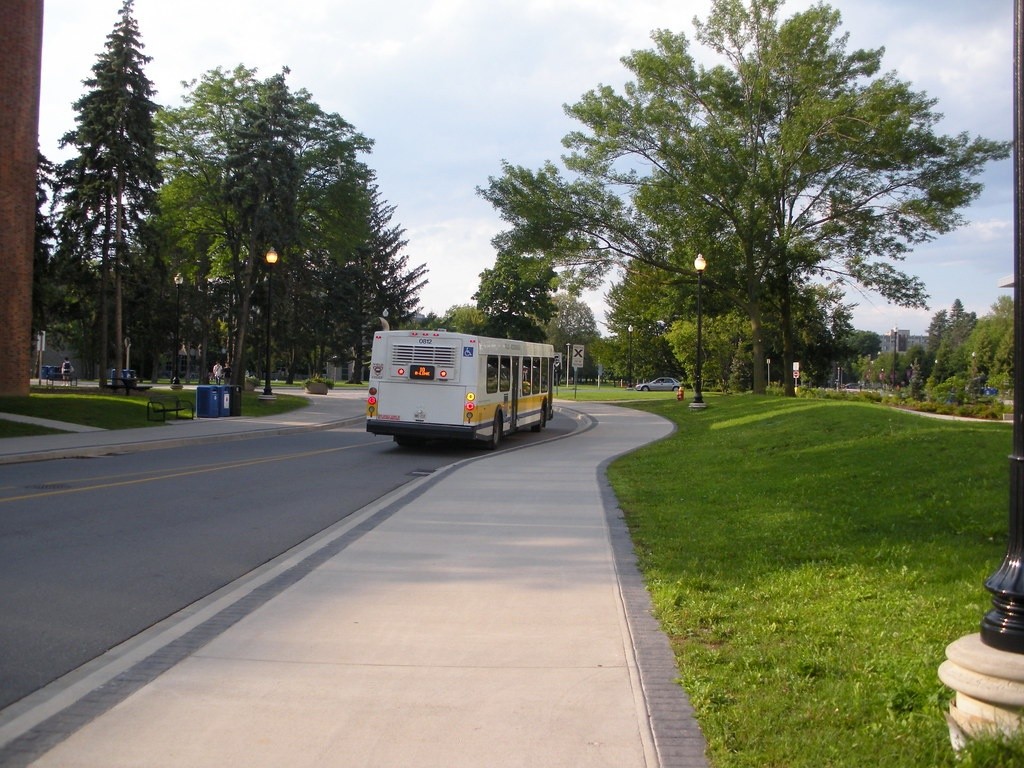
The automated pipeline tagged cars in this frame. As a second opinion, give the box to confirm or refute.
[635,377,681,391]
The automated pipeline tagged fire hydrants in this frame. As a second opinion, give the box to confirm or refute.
[676,386,684,401]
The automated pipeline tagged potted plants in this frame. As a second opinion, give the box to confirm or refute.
[303,376,335,396]
[243,374,261,392]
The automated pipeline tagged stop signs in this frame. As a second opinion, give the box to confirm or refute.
[793,370,800,378]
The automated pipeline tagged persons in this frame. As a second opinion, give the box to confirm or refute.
[61,357,75,386]
[222,363,231,385]
[213,361,222,385]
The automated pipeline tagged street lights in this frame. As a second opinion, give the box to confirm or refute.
[566,342,571,387]
[262,245,279,395]
[172,271,185,384]
[628,325,634,387]
[692,252,709,403]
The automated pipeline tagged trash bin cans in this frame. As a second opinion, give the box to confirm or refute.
[123,370,135,378]
[217,385,230,417]
[229,385,242,416]
[112,369,122,384]
[196,385,218,418]
[42,366,61,379]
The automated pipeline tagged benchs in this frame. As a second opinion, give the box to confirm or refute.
[147,395,196,424]
[102,384,153,394]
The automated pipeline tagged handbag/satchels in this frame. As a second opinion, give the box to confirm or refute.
[211,366,220,379]
[70,361,75,373]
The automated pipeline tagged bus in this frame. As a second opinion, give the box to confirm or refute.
[365,316,561,450]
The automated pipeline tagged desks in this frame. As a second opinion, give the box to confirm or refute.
[111,377,145,394]
